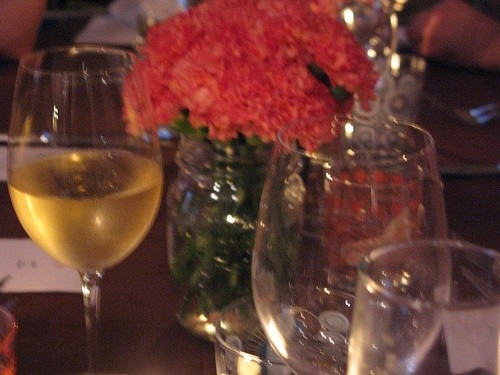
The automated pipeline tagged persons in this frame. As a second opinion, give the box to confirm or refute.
[404,0,500,72]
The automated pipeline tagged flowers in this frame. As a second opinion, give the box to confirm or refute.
[116,0,384,312]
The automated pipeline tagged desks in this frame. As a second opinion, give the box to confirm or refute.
[0,65,500,375]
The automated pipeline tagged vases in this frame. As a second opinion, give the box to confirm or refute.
[160,117,272,349]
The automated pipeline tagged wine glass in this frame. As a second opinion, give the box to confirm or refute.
[5,47,166,375]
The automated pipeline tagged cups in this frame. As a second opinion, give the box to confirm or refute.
[344,243,500,375]
[0,303,17,375]
[251,113,449,375]
[212,303,295,374]
